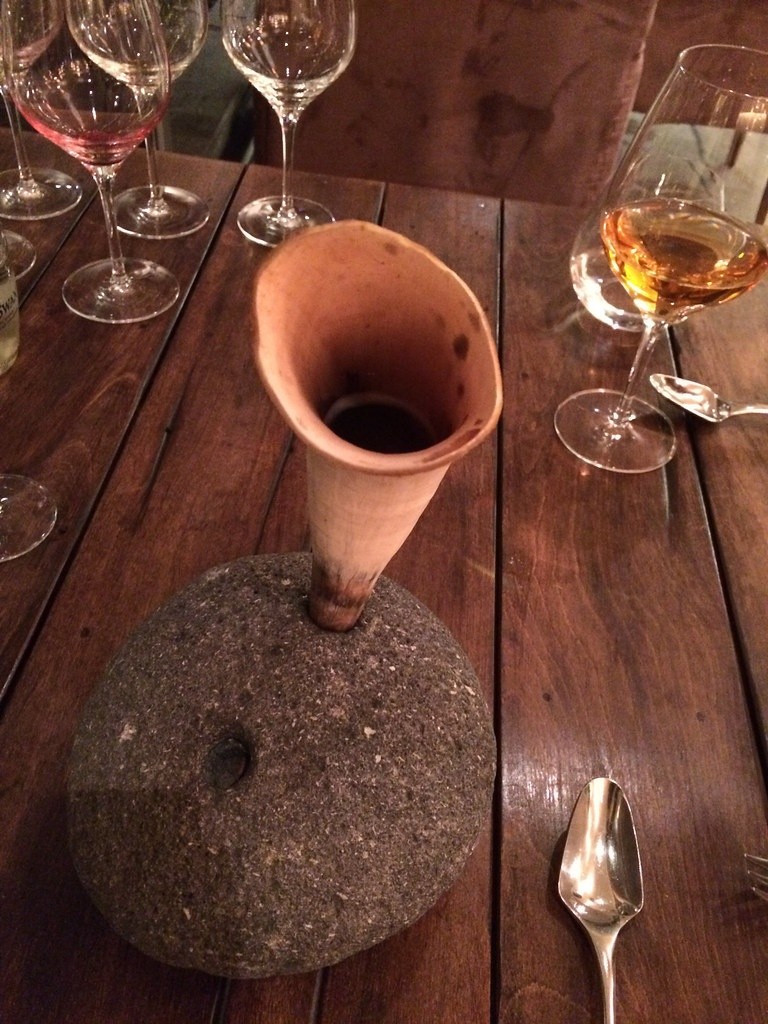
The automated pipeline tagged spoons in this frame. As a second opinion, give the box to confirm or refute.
[558,778,645,1024]
[649,373,768,423]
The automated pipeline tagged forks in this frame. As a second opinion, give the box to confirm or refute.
[744,853,768,902]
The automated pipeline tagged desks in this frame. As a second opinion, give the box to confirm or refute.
[0,124,767,1024]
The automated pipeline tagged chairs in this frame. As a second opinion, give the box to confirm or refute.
[254,1,767,226]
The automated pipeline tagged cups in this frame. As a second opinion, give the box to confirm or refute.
[569,156,725,332]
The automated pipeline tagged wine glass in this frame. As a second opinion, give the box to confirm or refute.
[553,44,768,473]
[66,0,210,240]
[0,1,82,220]
[0,474,57,563]
[220,1,356,249]
[0,0,180,323]
[0,225,37,279]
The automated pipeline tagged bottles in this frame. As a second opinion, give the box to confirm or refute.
[0,222,21,379]
